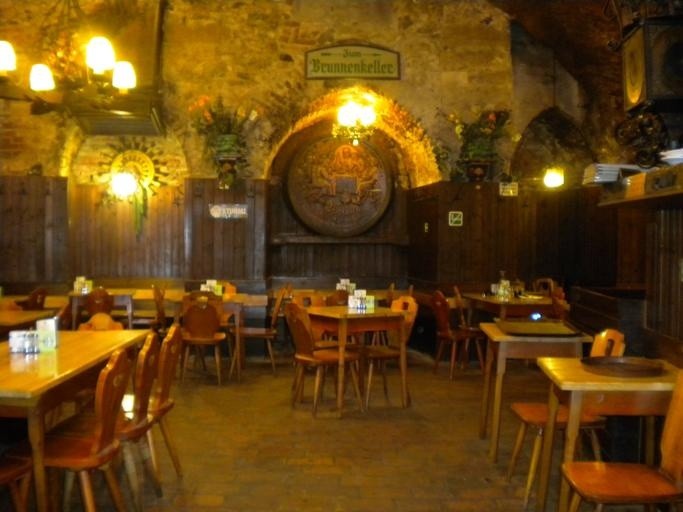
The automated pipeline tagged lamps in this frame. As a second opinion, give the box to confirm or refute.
[0,0,142,122]
[327,96,377,149]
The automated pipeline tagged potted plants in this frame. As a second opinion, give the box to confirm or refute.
[194,93,253,177]
[437,107,505,190]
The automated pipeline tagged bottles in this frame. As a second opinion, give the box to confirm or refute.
[8,330,38,354]
[356,296,367,309]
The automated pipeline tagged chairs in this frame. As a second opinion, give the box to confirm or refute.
[1,274,180,512]
[561,369,683,512]
[508,328,625,511]
[180,273,569,417]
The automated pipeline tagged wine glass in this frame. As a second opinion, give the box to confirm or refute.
[497,269,515,302]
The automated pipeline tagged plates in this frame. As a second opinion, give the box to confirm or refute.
[659,149,683,166]
[579,355,664,377]
[594,162,642,184]
[518,295,543,300]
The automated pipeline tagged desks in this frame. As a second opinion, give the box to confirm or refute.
[480,321,593,465]
[536,357,682,511]
